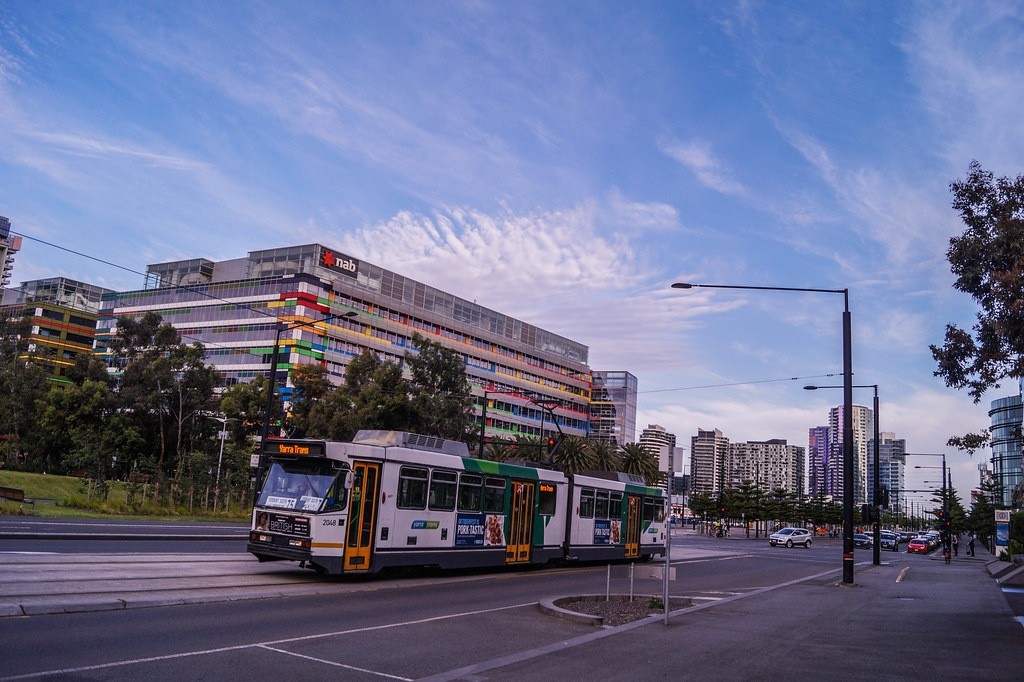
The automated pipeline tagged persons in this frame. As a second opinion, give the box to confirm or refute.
[829,529,834,539]
[724,524,728,537]
[832,529,839,539]
[952,535,958,557]
[966,533,975,557]
[943,536,951,564]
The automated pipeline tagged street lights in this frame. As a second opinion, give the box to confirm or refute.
[253,310,358,528]
[803,385,883,565]
[719,446,759,529]
[682,464,691,527]
[902,452,946,555]
[687,456,696,530]
[914,466,952,513]
[479,391,534,460]
[671,283,856,582]
[755,461,789,538]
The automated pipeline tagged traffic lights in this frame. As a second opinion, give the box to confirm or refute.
[720,503,725,513]
[939,511,944,521]
[547,438,556,454]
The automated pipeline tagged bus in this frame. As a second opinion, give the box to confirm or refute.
[246,429,669,576]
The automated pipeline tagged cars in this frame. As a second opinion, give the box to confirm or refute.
[880,533,899,552]
[907,539,928,555]
[853,534,871,549]
[769,528,813,549]
[864,528,943,552]
